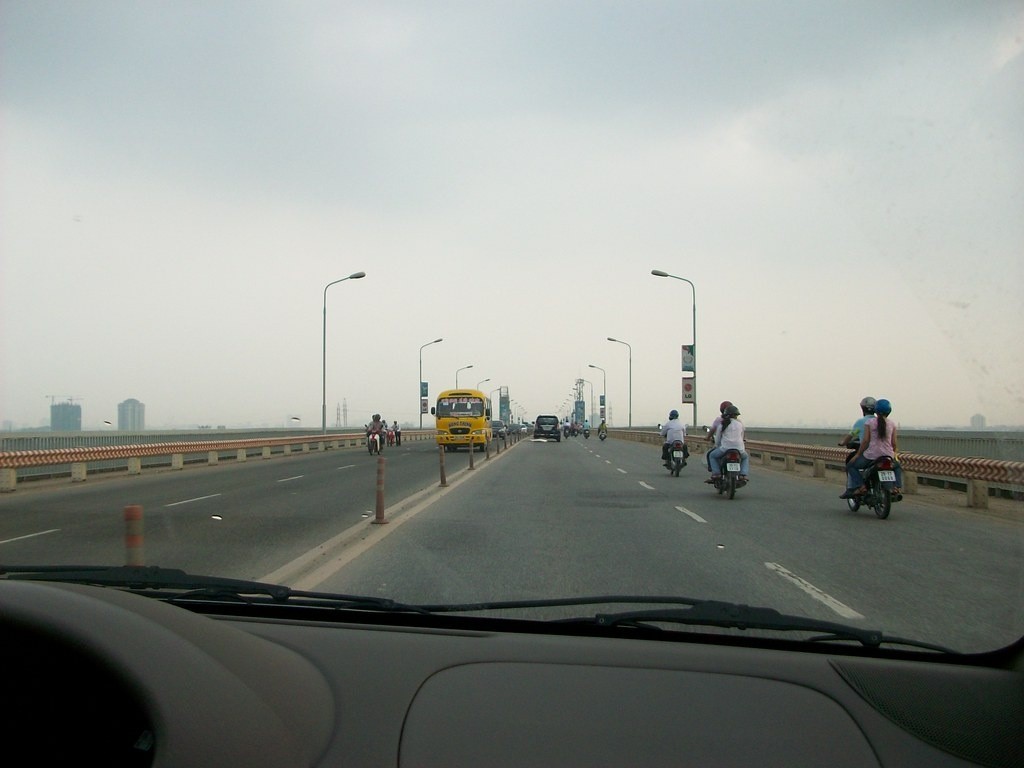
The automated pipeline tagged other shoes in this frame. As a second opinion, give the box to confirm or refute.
[662,464,667,466]
[711,474,721,479]
[839,492,853,499]
[684,462,687,465]
[704,479,712,483]
[740,475,750,482]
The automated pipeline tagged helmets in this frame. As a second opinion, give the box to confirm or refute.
[723,406,741,415]
[669,410,678,417]
[720,401,732,409]
[860,396,876,409]
[875,399,891,414]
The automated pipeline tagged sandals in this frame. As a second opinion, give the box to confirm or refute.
[892,487,899,495]
[851,487,867,495]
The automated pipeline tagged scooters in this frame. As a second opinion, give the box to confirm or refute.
[598,429,608,441]
[365,424,383,456]
[564,429,591,439]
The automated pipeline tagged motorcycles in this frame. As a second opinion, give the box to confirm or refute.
[837,436,903,519]
[658,424,690,476]
[702,425,746,500]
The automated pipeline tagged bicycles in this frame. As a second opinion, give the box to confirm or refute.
[386,429,396,446]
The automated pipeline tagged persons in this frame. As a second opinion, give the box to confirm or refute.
[382,419,402,447]
[704,401,750,484]
[839,397,902,503]
[563,418,607,436]
[367,414,385,451]
[659,410,690,465]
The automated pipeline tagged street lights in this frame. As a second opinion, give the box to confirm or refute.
[420,338,443,430]
[456,365,528,424]
[322,272,366,448]
[588,365,607,424]
[651,270,697,436]
[607,337,631,429]
[561,378,593,429]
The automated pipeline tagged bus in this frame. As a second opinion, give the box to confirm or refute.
[431,389,492,452]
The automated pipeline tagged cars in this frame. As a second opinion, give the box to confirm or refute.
[532,415,561,442]
[492,420,533,440]
